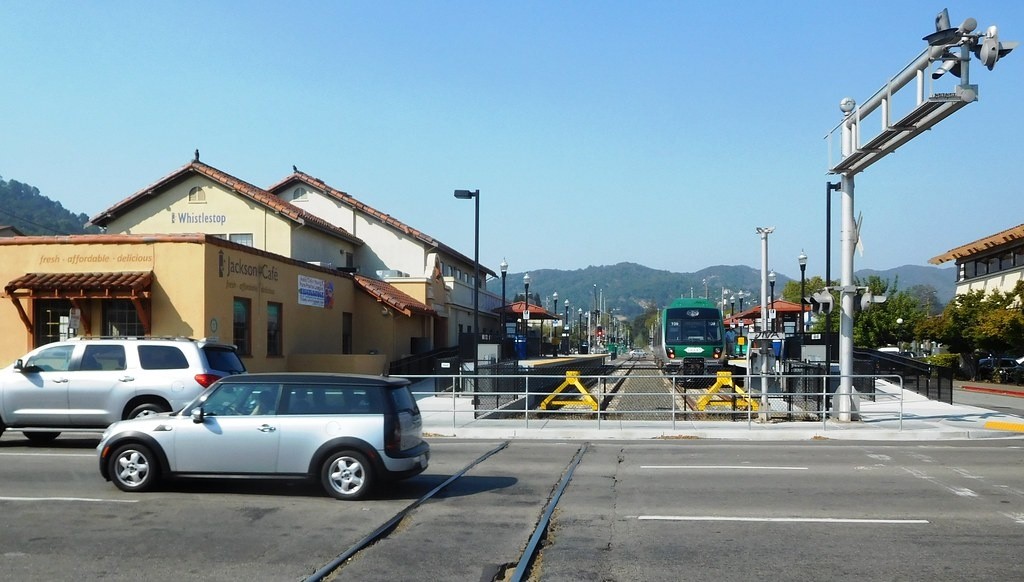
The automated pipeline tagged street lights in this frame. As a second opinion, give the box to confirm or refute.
[565,298,569,354]
[500,257,508,361]
[585,311,588,344]
[523,271,530,358]
[768,269,777,331]
[553,290,558,357]
[578,308,582,345]
[826,180,856,420]
[731,294,735,328]
[896,318,903,353]
[923,290,937,353]
[738,288,744,355]
[455,189,479,394]
[756,225,777,421]
[798,248,808,344]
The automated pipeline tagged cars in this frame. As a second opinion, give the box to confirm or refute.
[858,348,916,374]
[97,373,429,499]
[979,357,1024,380]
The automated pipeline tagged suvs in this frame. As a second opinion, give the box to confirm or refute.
[0,335,250,439]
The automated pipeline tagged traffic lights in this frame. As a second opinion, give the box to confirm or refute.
[802,292,833,314]
[598,326,602,336]
[855,288,887,314]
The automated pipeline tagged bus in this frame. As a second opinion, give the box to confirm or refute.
[652,296,727,379]
